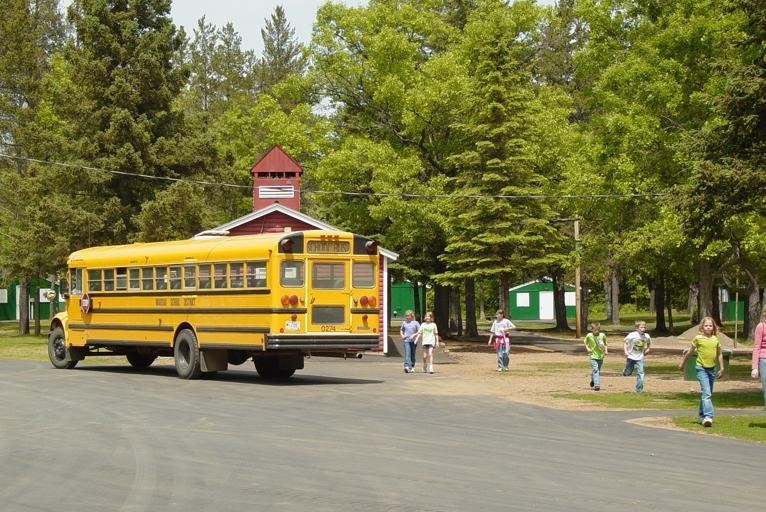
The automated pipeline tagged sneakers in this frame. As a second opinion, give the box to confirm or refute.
[404,367,414,373]
[698,417,712,426]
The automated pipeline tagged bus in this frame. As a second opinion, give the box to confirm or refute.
[48,230,380,379]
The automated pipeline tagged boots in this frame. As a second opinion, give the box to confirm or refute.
[423,364,434,374]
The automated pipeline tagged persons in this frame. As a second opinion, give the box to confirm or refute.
[678,317,725,427]
[584,321,608,391]
[751,304,766,406]
[622,320,652,392]
[399,310,421,373]
[488,309,516,372]
[414,311,439,374]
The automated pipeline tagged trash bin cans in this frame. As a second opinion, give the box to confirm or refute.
[684,351,731,381]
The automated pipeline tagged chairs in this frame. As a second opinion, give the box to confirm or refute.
[133,277,263,290]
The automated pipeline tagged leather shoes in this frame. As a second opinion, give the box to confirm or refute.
[590,381,600,391]
